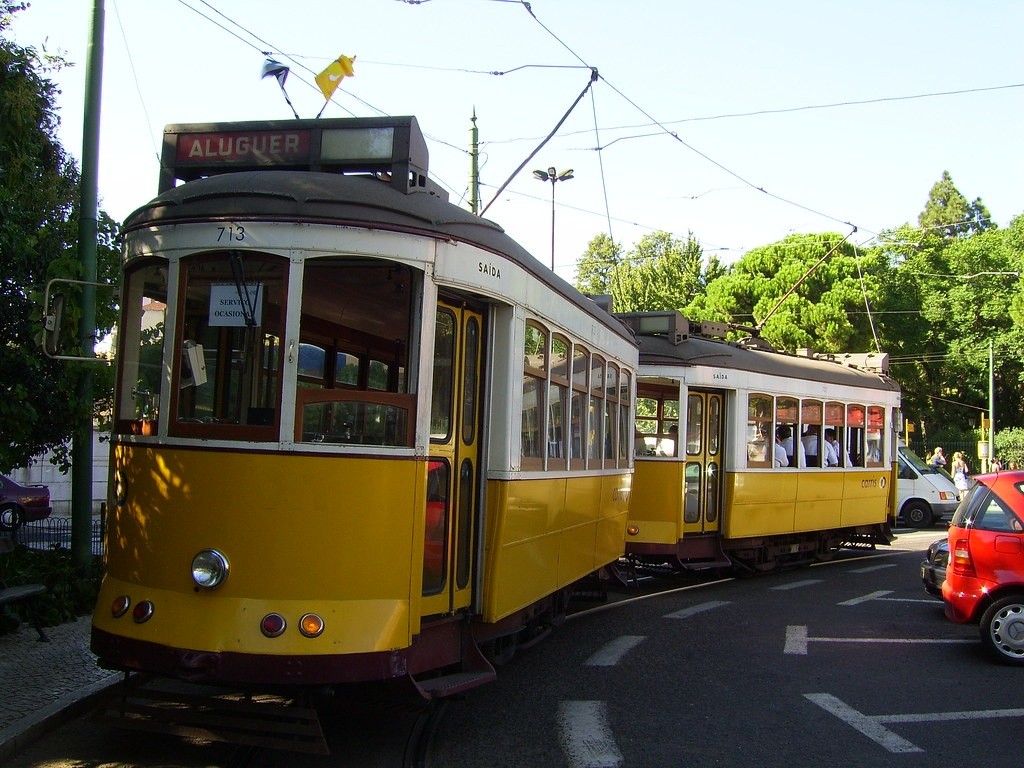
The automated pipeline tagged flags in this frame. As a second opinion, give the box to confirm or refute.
[260,59,289,88]
[314,54,356,101]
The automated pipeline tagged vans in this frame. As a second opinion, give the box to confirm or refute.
[865,433,963,529]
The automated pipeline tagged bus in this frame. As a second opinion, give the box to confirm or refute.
[41,64,641,724]
[580,223,903,595]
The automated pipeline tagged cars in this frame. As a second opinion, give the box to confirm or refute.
[940,469,1024,666]
[0,472,53,532]
[920,520,1008,600]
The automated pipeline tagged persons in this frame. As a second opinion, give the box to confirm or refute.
[991,457,1004,473]
[667,425,689,458]
[928,446,947,466]
[760,425,853,467]
[950,452,969,500]
[633,424,646,457]
